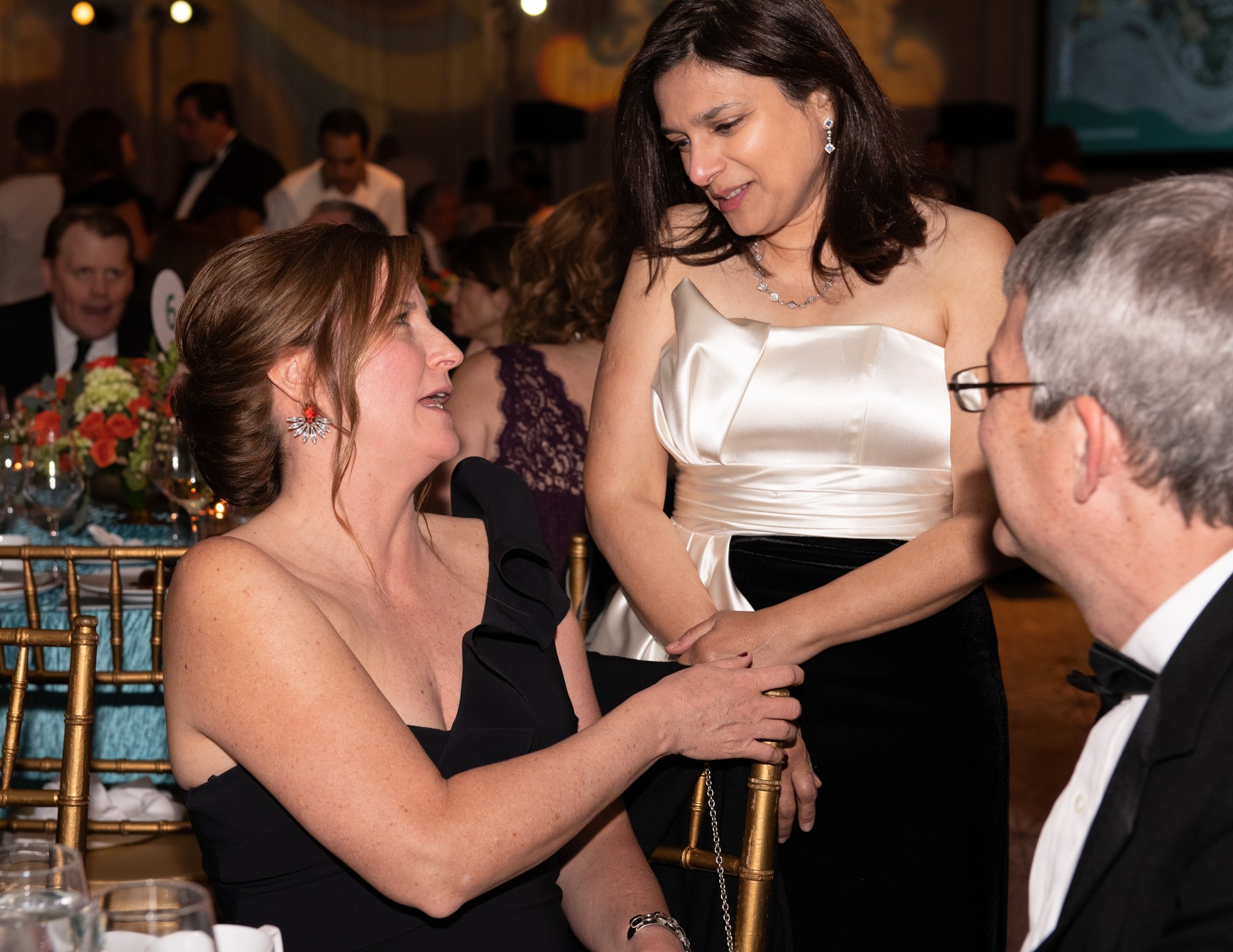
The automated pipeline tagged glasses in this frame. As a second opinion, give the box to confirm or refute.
[948,366,1041,413]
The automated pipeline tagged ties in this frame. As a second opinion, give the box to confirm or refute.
[72,340,92,372]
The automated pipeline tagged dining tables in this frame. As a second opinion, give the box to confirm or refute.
[0,491,196,784]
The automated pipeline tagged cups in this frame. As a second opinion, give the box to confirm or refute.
[0,842,218,952]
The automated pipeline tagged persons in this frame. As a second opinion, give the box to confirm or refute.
[164,224,804,951]
[264,110,408,231]
[1,205,162,414]
[62,106,168,300]
[173,80,286,231]
[0,108,65,413]
[949,171,1233,952]
[183,128,1090,600]
[582,0,1018,952]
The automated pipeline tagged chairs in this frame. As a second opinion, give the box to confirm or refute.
[0,546,789,951]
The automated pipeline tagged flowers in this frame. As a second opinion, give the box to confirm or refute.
[0,337,190,511]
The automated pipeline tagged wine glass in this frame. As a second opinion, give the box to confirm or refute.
[152,419,215,546]
[22,426,85,578]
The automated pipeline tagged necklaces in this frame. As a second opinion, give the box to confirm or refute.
[754,240,844,308]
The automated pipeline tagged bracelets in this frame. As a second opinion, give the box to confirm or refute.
[626,911,691,952]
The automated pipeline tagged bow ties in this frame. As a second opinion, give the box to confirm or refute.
[1067,641,1158,724]
